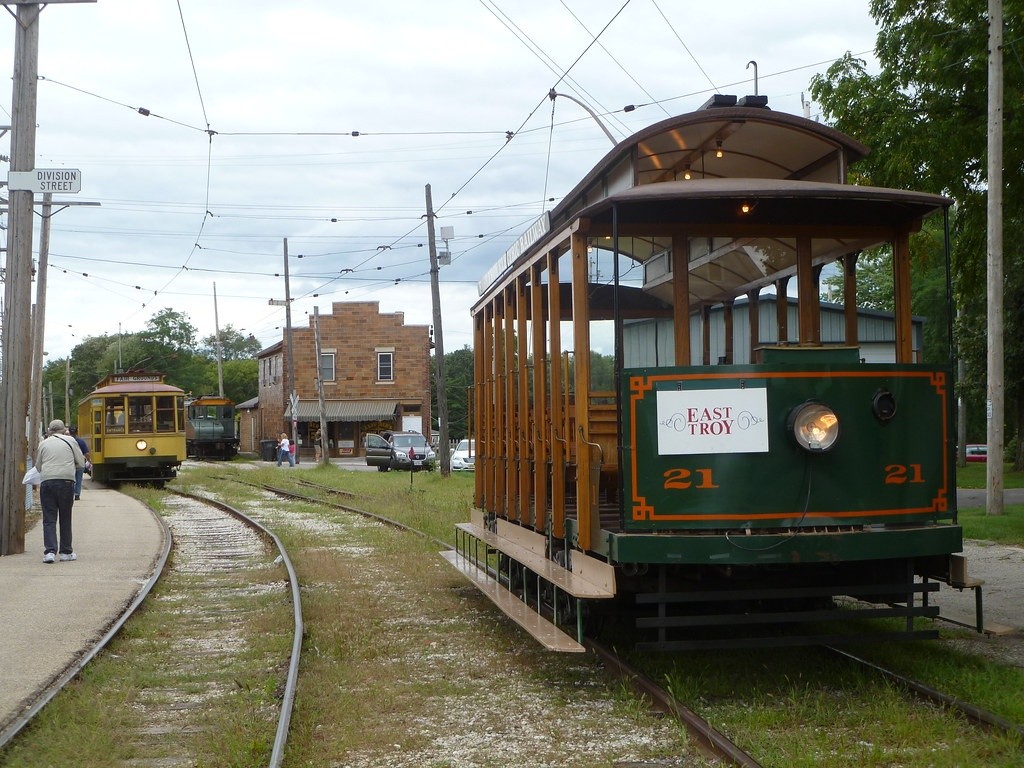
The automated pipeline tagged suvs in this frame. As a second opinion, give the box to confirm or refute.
[365,430,436,472]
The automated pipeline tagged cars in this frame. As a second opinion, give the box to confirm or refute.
[449,439,475,472]
[955,444,987,462]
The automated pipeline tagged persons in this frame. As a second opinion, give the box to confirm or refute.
[35,420,89,563]
[312,429,321,463]
[276,433,295,467]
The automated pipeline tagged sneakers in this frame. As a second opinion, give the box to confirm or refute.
[59,553,76,560]
[43,553,55,562]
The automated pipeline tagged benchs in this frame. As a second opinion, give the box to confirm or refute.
[475,391,623,505]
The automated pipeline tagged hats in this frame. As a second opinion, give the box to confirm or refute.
[69,426,77,432]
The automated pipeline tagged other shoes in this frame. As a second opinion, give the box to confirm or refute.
[75,496,80,500]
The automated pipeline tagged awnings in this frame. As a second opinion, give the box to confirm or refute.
[283,401,397,422]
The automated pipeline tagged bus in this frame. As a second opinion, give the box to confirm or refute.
[184,391,240,461]
[437,94,1014,654]
[78,372,186,488]
